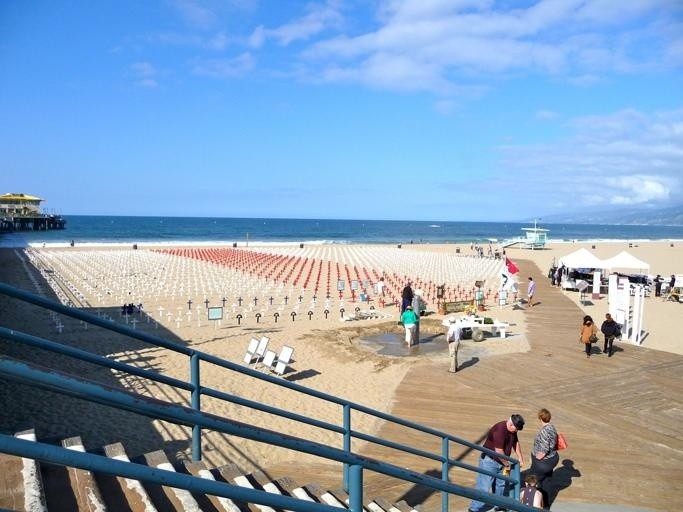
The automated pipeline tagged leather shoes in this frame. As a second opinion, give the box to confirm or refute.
[495,507,506,512]
[468,508,473,511]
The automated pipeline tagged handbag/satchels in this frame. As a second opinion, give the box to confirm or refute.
[589,334,598,343]
[557,432,568,449]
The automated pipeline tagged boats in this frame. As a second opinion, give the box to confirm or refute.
[0,192,66,230]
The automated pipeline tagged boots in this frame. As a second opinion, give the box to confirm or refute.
[603,344,607,353]
[608,348,612,357]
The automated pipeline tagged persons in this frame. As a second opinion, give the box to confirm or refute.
[550,265,565,288]
[581,315,596,357]
[654,275,664,296]
[669,275,675,288]
[403,283,413,310]
[377,277,385,308]
[468,414,525,512]
[471,241,492,255]
[531,409,559,481]
[400,305,419,348]
[601,313,617,357]
[447,318,460,373]
[520,475,544,509]
[527,277,536,307]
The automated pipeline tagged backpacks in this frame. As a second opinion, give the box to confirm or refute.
[605,324,615,336]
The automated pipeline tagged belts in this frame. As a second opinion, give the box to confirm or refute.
[487,454,504,466]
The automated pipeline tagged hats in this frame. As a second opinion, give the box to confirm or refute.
[511,414,524,430]
[407,306,412,309]
[447,316,456,322]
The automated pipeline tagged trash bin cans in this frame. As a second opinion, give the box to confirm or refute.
[671,244,674,247]
[456,247,460,253]
[133,245,137,250]
[629,244,632,247]
[398,245,401,248]
[233,243,237,247]
[300,244,303,248]
[592,246,595,249]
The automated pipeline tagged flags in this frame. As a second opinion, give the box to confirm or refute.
[506,256,519,274]
